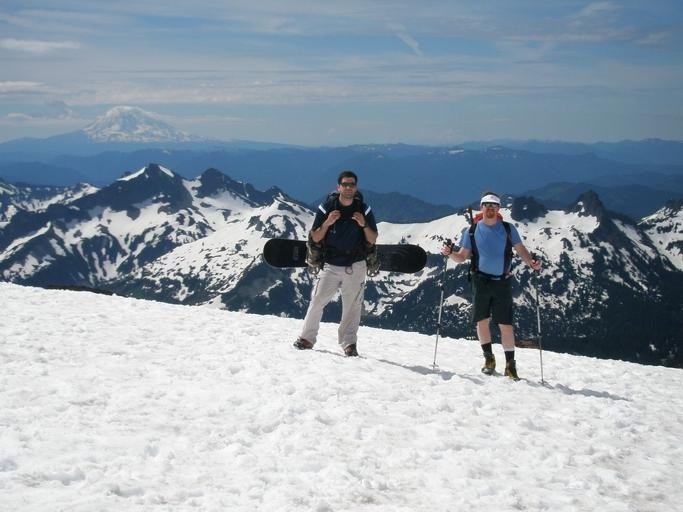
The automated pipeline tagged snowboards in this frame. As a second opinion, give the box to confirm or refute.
[263,239,427,273]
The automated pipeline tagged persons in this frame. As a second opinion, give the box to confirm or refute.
[436,189,542,384]
[289,169,380,357]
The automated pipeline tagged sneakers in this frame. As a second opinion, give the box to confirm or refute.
[293,337,312,350]
[344,343,357,356]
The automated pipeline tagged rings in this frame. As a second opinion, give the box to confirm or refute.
[445,250,449,252]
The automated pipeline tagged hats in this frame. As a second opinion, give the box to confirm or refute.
[479,194,501,207]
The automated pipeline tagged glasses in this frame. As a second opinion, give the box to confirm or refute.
[338,182,357,187]
[483,203,499,208]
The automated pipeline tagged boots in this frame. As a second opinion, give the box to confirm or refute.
[482,353,496,376]
[504,359,520,382]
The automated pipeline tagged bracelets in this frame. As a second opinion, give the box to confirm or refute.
[529,263,534,270]
[446,248,452,257]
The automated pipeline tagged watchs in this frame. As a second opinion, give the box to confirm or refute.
[359,221,369,229]
